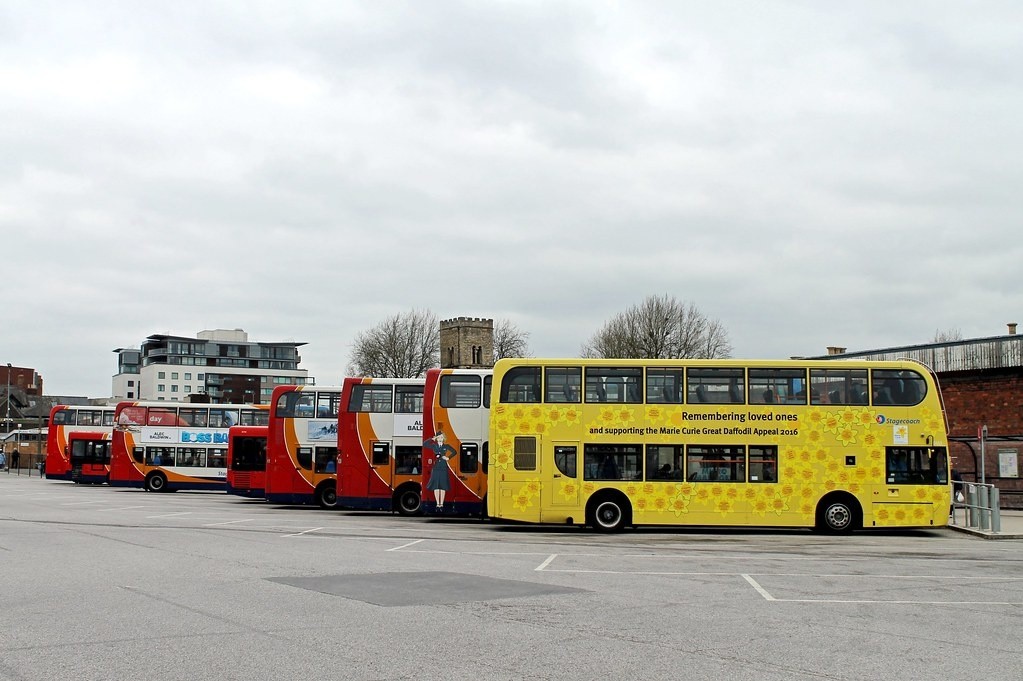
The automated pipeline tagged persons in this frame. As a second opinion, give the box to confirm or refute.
[221,417,230,428]
[11,448,19,468]
[0,449,7,472]
[948,462,963,518]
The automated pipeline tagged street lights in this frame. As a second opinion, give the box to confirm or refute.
[6,362,12,437]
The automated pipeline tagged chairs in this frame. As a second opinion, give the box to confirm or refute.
[889,464,903,481]
[531,381,920,406]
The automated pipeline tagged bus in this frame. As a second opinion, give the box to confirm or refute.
[418,369,681,520]
[43,405,118,485]
[227,425,268,501]
[335,376,541,519]
[487,356,953,536]
[264,384,424,511]
[109,401,332,494]
[1,428,51,468]
